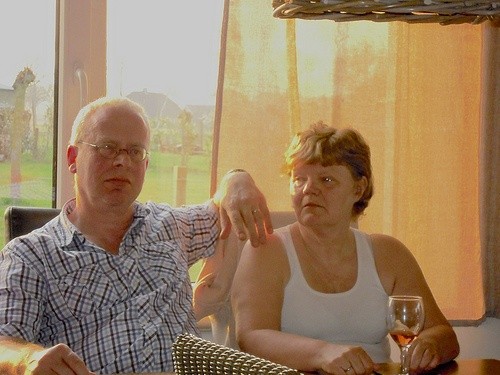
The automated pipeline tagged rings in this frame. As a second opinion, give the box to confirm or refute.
[344,366,353,373]
[252,209,261,214]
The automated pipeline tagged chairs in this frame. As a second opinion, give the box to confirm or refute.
[208,208,359,355]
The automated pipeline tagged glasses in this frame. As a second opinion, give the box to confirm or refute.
[79,142,151,161]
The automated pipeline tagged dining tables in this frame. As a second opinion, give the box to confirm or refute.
[113,358,500,375]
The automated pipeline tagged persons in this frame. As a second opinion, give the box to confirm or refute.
[231,120,460,375]
[0,96,274,375]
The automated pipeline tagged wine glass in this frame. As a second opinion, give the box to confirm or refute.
[386,295,425,375]
